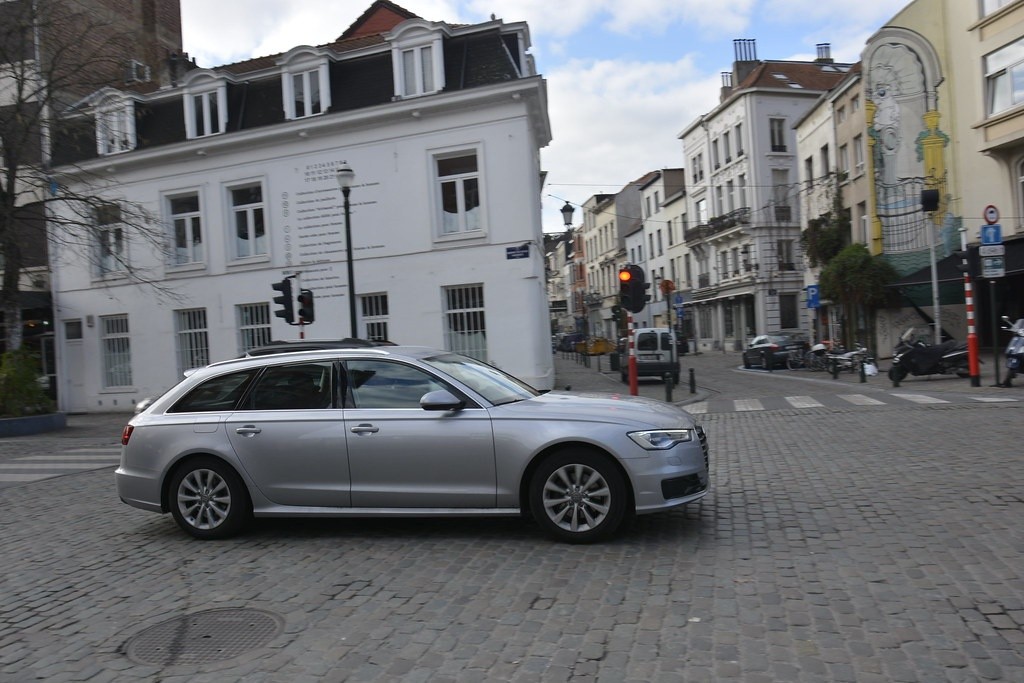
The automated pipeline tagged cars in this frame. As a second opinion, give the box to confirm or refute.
[743,330,811,371]
[667,331,689,355]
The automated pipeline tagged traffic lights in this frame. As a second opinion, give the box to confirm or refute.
[635,265,652,313]
[271,279,293,322]
[619,266,637,312]
[297,289,315,322]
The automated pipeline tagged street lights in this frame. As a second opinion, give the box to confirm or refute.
[335,159,359,338]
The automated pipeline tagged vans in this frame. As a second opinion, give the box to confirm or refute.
[620,327,680,385]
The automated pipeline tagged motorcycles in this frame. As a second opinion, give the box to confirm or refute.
[827,341,880,375]
[888,327,986,381]
[1000,315,1024,387]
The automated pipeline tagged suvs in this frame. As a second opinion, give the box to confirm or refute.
[113,336,715,543]
